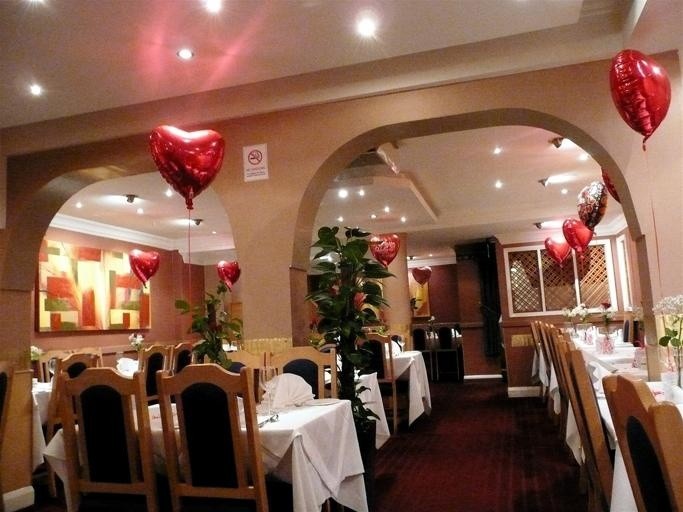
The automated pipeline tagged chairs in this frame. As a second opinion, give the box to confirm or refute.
[57,366,157,512]
[170,342,194,403]
[205,351,265,403]
[139,344,170,406]
[355,334,408,434]
[602,373,683,511]
[35,352,98,498]
[530,321,613,512]
[623,312,634,343]
[265,346,344,512]
[36,349,72,383]
[156,365,270,511]
[412,324,433,381]
[72,347,102,367]
[432,324,460,381]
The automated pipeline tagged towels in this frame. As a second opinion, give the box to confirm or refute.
[261,373,315,410]
[117,357,138,375]
[385,340,401,357]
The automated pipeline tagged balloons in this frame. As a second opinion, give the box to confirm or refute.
[562,218,593,259]
[602,169,623,203]
[545,237,571,267]
[217,260,241,292]
[577,181,608,235]
[609,49,672,152]
[370,233,400,268]
[412,266,432,288]
[128,248,160,288]
[147,125,224,209]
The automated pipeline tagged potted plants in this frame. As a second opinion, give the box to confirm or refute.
[303,226,398,458]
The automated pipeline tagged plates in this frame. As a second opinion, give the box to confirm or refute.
[306,398,340,406]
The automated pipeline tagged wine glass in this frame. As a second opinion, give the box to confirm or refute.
[597,327,619,353]
[259,366,277,416]
[114,351,123,366]
[577,323,593,345]
[397,335,406,353]
[49,359,56,374]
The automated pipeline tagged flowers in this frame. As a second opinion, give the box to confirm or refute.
[127,334,144,350]
[600,302,616,327]
[652,295,683,386]
[572,303,589,323]
